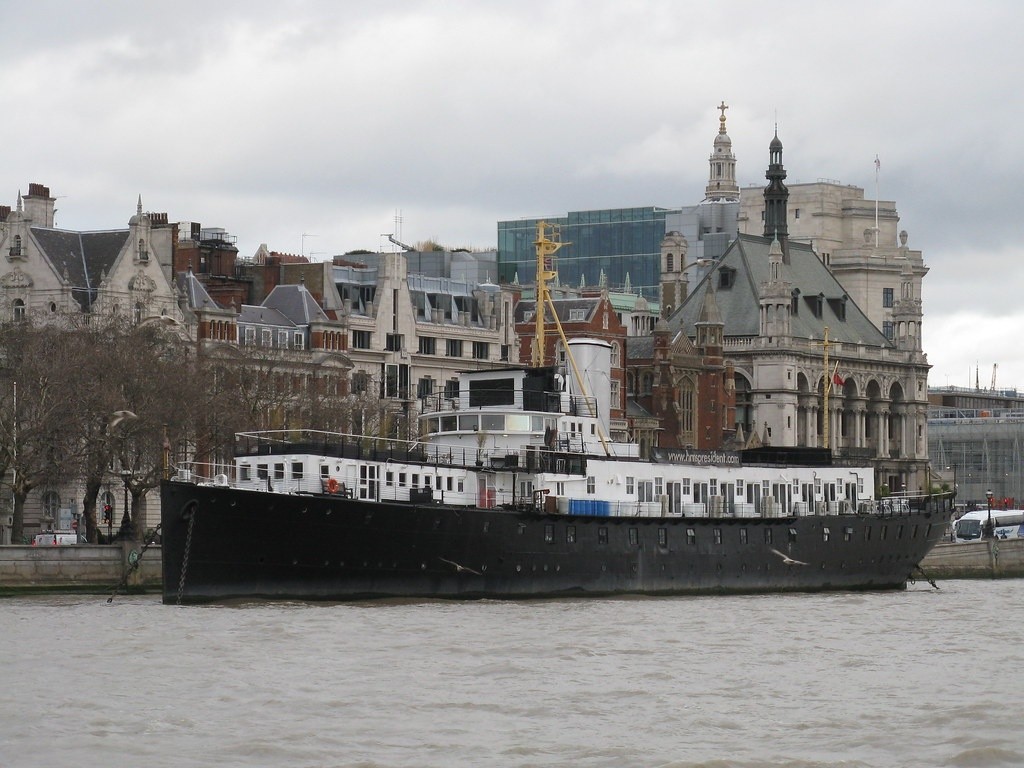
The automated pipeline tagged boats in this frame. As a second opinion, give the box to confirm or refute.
[161,222,956,603]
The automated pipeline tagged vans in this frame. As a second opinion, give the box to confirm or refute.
[33,530,86,544]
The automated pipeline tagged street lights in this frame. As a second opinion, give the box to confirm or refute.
[984,489,994,537]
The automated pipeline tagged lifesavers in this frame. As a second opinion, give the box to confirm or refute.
[327,479,338,494]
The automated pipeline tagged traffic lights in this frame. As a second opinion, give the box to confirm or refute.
[104,504,110,524]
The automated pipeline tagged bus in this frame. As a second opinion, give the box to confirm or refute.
[952,509,1024,542]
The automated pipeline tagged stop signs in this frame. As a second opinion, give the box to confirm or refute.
[73,522,77,529]
